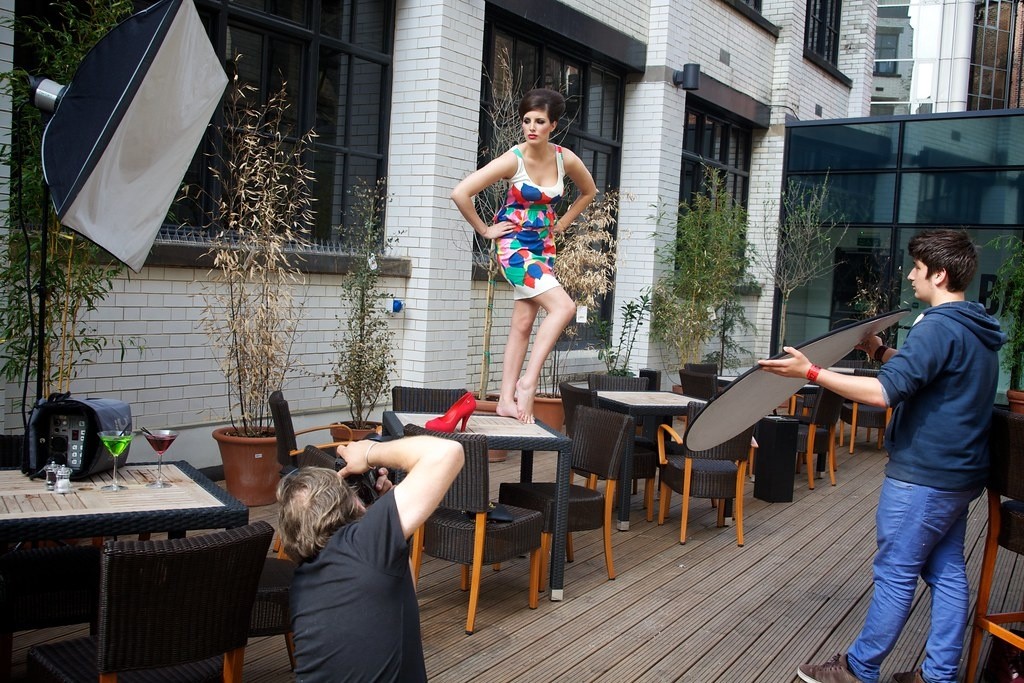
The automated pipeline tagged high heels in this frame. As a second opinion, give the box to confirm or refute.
[425,391,477,433]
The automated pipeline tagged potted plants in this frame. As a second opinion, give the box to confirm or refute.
[531,186,634,432]
[643,152,759,423]
[749,162,851,409]
[176,54,320,507]
[321,175,408,459]
[983,234,1024,414]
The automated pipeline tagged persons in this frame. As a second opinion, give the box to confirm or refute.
[273,435,465,683]
[758,228,1009,683]
[451,89,597,424]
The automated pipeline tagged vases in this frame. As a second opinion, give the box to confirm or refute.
[471,393,506,462]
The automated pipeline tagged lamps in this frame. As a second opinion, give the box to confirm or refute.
[673,63,700,92]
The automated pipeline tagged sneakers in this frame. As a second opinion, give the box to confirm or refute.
[891,664,925,683]
[796,652,861,683]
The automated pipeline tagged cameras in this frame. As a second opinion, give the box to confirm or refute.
[335,433,393,505]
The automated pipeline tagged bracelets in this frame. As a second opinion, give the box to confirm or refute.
[807,364,821,382]
[875,345,890,362]
[366,442,380,470]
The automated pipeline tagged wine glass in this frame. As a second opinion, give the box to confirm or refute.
[142,430,179,488]
[98,431,136,491]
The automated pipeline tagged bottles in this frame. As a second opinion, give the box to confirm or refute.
[54,464,72,493]
[44,461,61,489]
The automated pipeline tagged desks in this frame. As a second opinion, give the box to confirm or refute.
[0,459,249,683]
[718,376,827,480]
[597,390,735,527]
[827,366,855,376]
[382,409,573,602]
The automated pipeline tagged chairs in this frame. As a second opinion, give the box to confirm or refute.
[962,404,1024,683]
[29,363,891,683]
